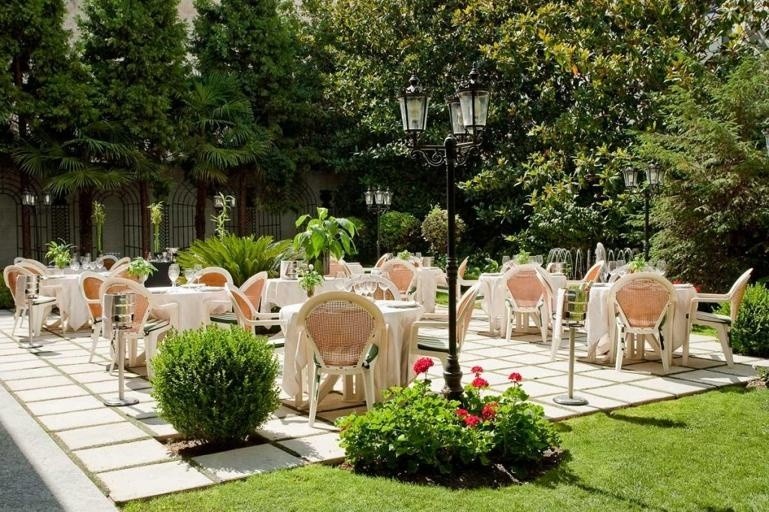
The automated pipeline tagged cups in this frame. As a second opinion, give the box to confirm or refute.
[397,251,422,267]
[606,258,670,277]
[421,255,435,266]
[501,253,544,267]
[551,263,567,275]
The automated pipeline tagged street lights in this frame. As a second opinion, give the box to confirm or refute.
[399,61,493,402]
[621,158,664,261]
[212,193,236,216]
[19,190,53,262]
[362,185,394,261]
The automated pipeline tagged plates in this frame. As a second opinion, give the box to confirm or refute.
[149,289,166,294]
[48,275,64,278]
[673,283,695,289]
[482,273,504,277]
[549,272,563,276]
[592,283,613,288]
[201,286,226,293]
[387,302,418,309]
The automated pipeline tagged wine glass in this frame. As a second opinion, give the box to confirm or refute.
[68,252,105,275]
[334,269,393,304]
[167,261,205,289]
[156,248,178,262]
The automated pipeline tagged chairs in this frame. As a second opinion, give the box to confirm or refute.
[682,267,754,369]
[79,271,482,425]
[587,275,695,373]
[5,253,604,348]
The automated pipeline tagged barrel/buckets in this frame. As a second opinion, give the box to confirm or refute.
[111,293,135,329]
[23,274,40,300]
